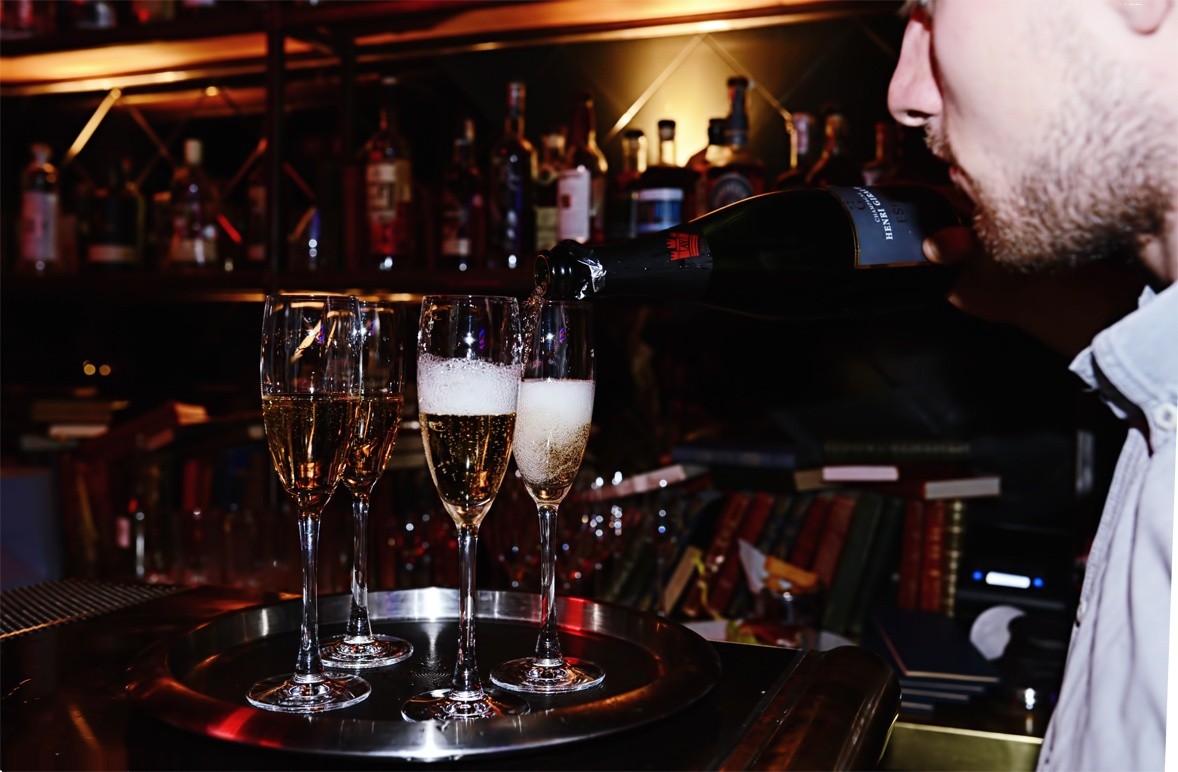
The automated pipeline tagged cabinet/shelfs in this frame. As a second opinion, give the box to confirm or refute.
[0,0,940,458]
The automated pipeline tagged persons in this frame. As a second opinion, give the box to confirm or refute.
[887,0,1178,772]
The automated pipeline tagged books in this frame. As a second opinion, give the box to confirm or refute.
[592,490,976,637]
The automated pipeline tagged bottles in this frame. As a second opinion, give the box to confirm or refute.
[533,186,967,320]
[1,0,905,274]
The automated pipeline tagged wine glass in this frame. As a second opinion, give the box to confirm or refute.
[315,297,414,669]
[488,300,605,695]
[245,290,373,715]
[401,293,531,725]
